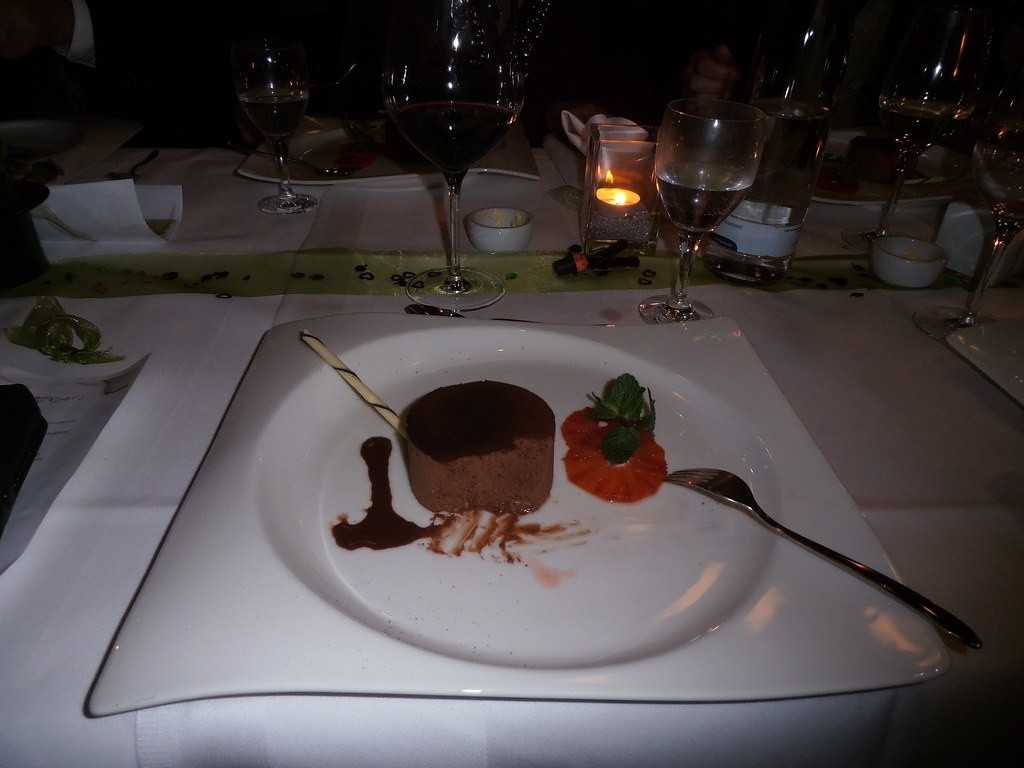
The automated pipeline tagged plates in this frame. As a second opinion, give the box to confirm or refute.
[235,113,542,186]
[87,310,952,719]
[811,126,954,207]
[947,320,1024,411]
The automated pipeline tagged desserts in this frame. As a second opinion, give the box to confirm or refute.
[303,328,557,517]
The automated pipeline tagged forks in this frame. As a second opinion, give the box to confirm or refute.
[104,149,159,182]
[664,467,984,650]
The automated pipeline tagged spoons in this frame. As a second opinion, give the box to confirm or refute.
[222,137,360,178]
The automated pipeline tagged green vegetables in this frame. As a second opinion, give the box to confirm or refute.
[587,371,655,465]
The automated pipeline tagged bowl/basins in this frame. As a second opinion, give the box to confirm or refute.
[464,206,534,253]
[868,236,945,288]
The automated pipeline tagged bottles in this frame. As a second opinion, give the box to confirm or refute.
[702,1,854,290]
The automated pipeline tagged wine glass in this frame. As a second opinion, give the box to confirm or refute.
[839,0,995,246]
[911,73,1024,346]
[381,1,525,311]
[637,99,766,326]
[232,40,318,216]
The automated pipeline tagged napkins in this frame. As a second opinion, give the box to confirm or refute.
[561,110,644,157]
[30,178,183,256]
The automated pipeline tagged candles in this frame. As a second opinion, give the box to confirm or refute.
[595,184,643,221]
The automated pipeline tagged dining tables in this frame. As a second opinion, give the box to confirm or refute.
[0,109,1024,768]
